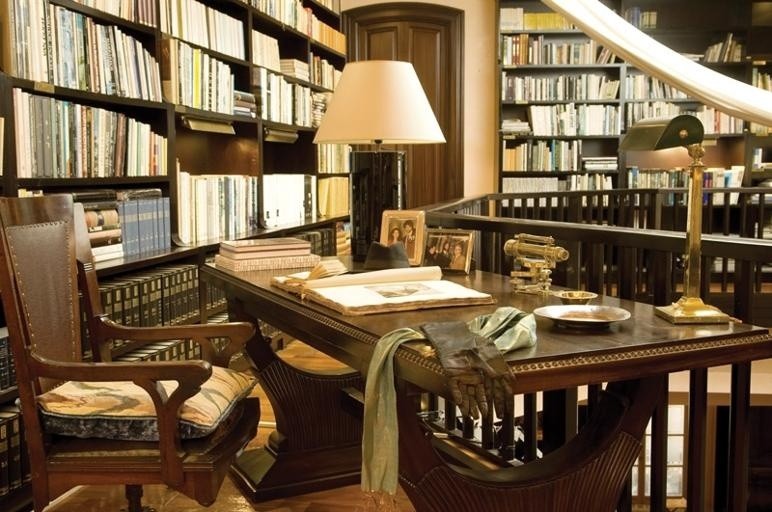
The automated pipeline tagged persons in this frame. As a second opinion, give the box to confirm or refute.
[426,240,465,268]
[386,220,416,259]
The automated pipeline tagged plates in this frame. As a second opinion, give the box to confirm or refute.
[531,305,631,327]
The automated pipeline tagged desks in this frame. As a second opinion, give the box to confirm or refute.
[192,254,772,510]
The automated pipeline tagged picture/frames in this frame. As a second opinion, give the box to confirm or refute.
[376,209,427,268]
[420,226,476,276]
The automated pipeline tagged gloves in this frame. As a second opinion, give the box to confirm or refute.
[422,319,499,422]
[472,330,518,420]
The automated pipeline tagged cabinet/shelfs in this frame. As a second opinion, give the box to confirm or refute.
[0,0,352,512]
[492,0,772,282]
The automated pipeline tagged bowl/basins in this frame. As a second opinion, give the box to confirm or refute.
[552,290,598,305]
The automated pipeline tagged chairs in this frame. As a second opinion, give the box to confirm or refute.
[0,190,264,510]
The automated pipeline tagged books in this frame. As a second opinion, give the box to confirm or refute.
[0,2,350,512]
[271,266,496,317]
[498,0,772,208]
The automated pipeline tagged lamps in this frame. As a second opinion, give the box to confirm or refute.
[307,58,450,264]
[617,112,732,329]
